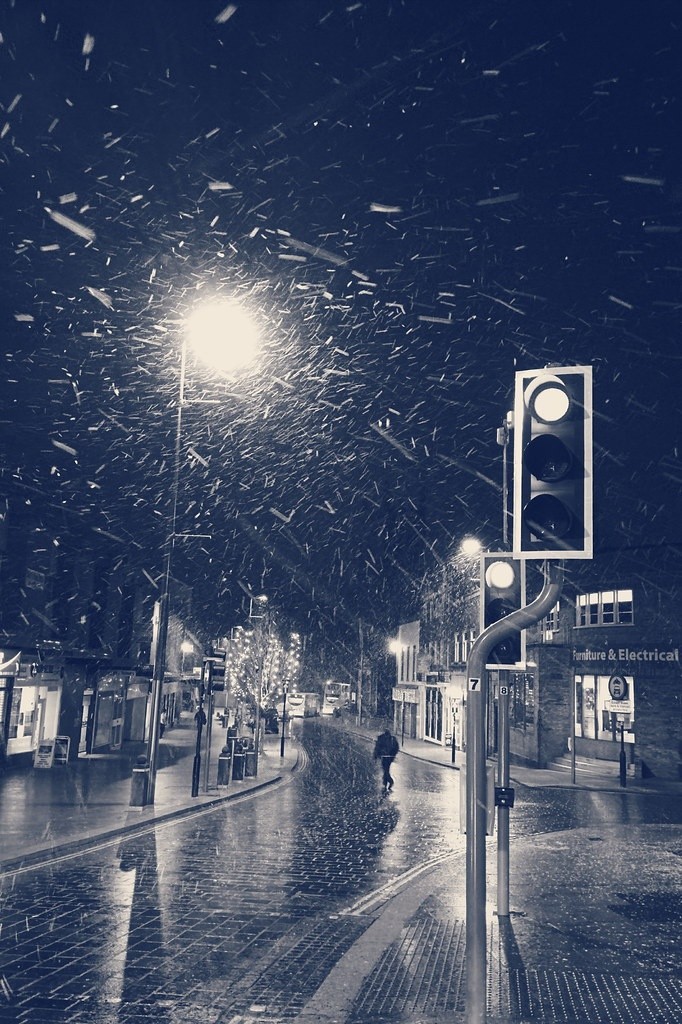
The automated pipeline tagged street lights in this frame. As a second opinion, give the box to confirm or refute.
[146,299,246,800]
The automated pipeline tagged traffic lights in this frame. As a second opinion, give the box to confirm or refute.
[479,553,528,671]
[510,365,593,559]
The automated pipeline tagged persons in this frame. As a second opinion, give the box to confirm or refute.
[332,708,339,717]
[194,708,206,729]
[160,709,168,738]
[373,728,399,789]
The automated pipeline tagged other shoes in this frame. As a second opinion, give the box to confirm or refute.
[381,784,387,792]
[388,781,395,789]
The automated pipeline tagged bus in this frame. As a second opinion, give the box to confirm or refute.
[284,692,320,718]
[322,682,350,713]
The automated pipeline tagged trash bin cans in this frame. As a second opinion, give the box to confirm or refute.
[221,714,229,728]
[216,746,232,786]
[232,745,245,780]
[128,754,150,807]
[245,743,257,779]
[445,733,452,746]
